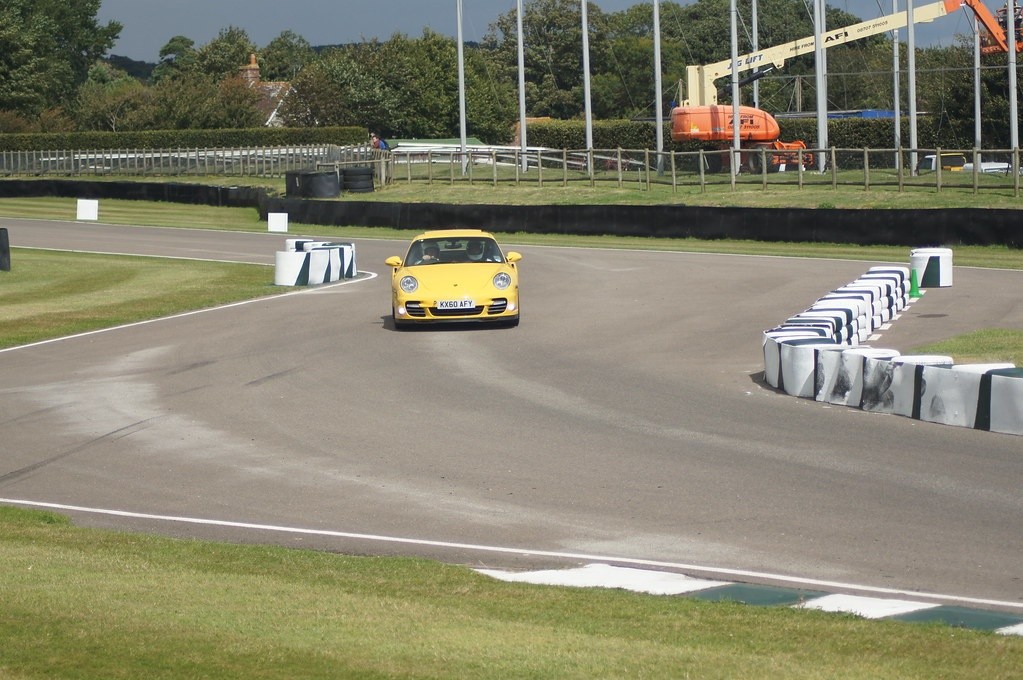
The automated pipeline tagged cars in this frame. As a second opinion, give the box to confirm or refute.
[917,155,937,170]
[385,229,523,329]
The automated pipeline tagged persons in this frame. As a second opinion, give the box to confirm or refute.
[413,241,442,265]
[462,240,488,263]
[369,131,390,151]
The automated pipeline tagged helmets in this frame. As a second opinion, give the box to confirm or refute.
[422,241,440,258]
[466,240,485,260]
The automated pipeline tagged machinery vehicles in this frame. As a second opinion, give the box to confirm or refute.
[667,0,1022,177]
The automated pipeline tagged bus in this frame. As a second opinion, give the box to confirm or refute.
[939,153,967,171]
